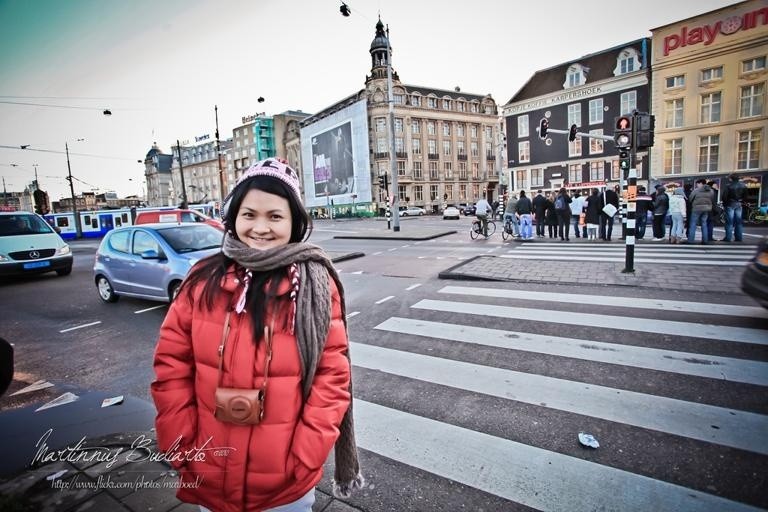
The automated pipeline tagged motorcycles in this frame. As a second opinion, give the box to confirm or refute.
[714,201,767,226]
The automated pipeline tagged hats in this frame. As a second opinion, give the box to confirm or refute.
[219,159,304,226]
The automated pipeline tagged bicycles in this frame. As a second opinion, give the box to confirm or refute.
[469,209,496,240]
[500,215,521,241]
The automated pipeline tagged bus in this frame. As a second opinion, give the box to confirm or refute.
[38,203,218,242]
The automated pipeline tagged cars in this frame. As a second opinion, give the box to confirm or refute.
[91,220,225,308]
[739,235,767,309]
[398,206,426,217]
[462,205,476,216]
[442,206,460,220]
[0,209,74,283]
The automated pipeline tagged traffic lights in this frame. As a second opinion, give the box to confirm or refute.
[634,111,654,150]
[612,114,633,149]
[568,123,576,141]
[617,148,630,170]
[378,175,386,190]
[540,118,549,138]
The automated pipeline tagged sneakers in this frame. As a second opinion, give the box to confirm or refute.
[515,233,743,244]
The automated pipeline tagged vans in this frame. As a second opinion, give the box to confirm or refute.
[133,208,226,233]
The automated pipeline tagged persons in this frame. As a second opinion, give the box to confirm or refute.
[150,156,365,512]
[475,173,748,244]
[335,128,348,185]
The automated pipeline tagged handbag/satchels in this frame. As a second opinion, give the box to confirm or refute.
[579,213,586,224]
[602,203,618,218]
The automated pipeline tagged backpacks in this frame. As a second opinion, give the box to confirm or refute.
[554,194,568,212]
